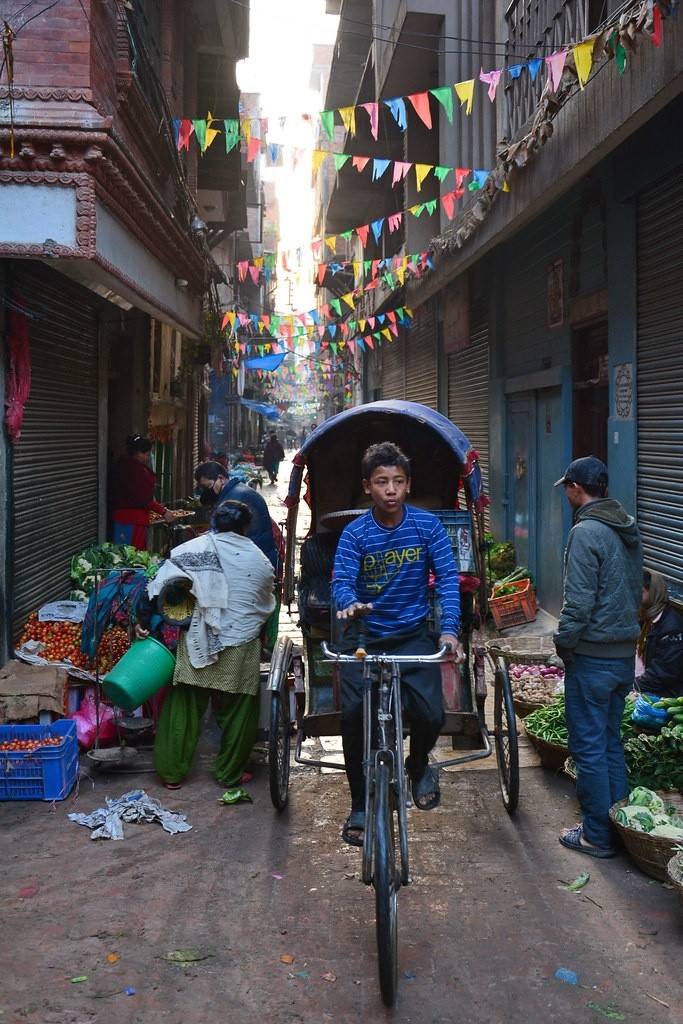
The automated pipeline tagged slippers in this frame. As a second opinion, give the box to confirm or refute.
[342,809,374,845]
[217,768,254,787]
[558,824,624,858]
[164,780,183,789]
[410,760,440,809]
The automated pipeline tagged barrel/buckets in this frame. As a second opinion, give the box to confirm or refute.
[101,634,176,713]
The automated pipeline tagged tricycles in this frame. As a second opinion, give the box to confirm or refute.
[266,397,522,1009]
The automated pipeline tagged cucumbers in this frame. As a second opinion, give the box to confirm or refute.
[642,694,683,728]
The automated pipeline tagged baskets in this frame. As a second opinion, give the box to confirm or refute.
[485,634,578,789]
[608,789,683,910]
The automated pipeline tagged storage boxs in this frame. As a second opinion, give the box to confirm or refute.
[0,719,79,801]
[487,579,537,630]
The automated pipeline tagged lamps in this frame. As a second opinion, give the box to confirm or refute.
[191,216,209,238]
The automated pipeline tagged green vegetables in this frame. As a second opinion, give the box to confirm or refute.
[482,533,536,603]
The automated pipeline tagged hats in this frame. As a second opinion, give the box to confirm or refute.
[553,453,610,489]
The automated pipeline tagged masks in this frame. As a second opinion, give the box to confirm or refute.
[204,478,223,501]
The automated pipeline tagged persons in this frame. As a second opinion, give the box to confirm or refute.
[330,442,466,846]
[108,432,177,556]
[128,500,276,790]
[632,570,683,699]
[553,455,643,858]
[194,461,285,662]
[263,424,317,488]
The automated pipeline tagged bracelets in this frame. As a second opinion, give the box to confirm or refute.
[161,509,168,520]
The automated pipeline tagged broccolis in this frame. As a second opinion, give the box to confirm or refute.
[615,785,683,840]
[70,547,128,603]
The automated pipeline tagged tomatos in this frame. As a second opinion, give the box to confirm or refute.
[15,611,135,678]
[0,736,64,761]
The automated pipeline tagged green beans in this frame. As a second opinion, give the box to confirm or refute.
[523,691,639,744]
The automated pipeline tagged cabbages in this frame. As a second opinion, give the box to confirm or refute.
[100,541,162,567]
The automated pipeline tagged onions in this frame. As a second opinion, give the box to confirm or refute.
[509,663,565,680]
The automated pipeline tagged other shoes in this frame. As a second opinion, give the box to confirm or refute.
[269,475,278,485]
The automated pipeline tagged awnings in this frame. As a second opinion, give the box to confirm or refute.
[240,398,281,422]
